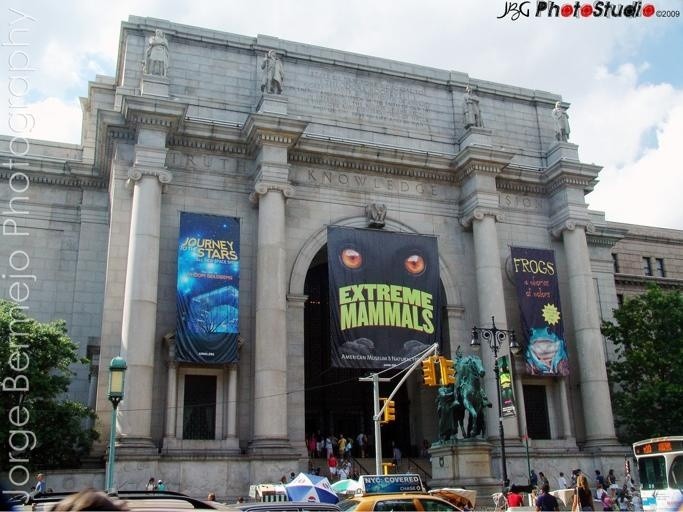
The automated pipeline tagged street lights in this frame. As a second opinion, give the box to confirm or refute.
[102,353,129,492]
[468,314,521,493]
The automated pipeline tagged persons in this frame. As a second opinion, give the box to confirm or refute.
[0,471,246,512]
[278,429,645,511]
[435,346,493,443]
[462,85,483,129]
[259,49,284,94]
[551,101,570,142]
[143,28,169,76]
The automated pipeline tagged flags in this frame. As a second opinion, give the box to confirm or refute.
[174,211,240,364]
[326,226,441,370]
[509,246,570,378]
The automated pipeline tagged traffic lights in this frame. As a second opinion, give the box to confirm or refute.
[419,355,436,387]
[437,355,456,387]
[383,399,396,422]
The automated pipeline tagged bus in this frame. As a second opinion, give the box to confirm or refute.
[631,435,682,511]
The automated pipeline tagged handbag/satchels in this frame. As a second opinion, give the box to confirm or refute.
[575,486,583,512]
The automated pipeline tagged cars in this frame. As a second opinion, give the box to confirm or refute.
[237,500,341,511]
[338,471,466,511]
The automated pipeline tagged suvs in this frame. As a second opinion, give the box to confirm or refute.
[3,488,242,512]
